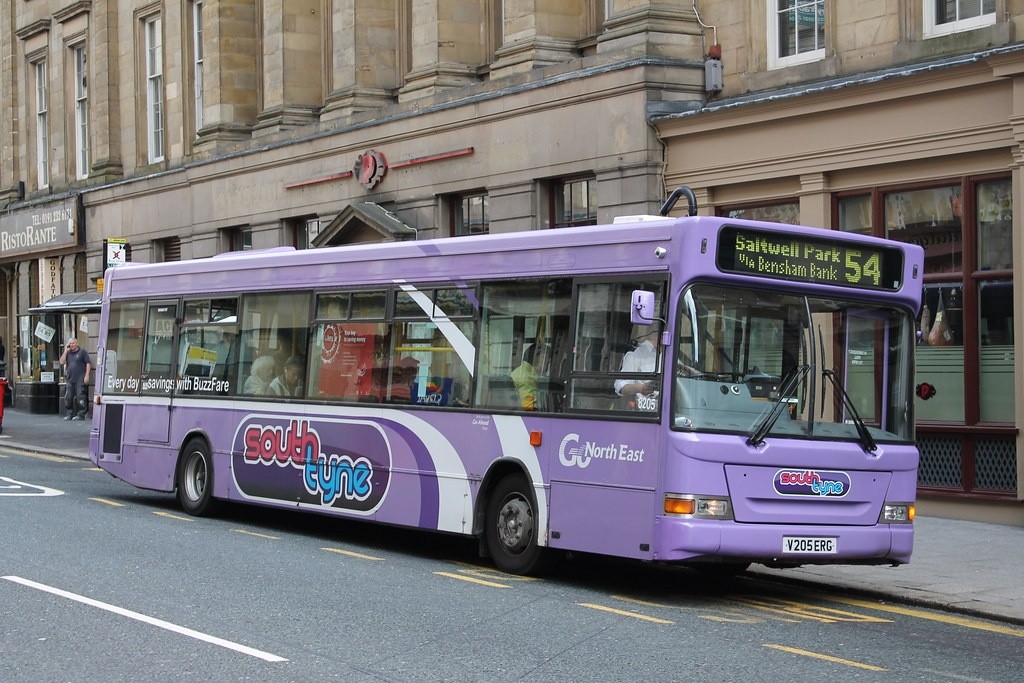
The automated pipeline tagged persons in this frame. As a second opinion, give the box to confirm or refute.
[387,357,432,404]
[614,313,707,411]
[267,355,309,397]
[244,355,279,396]
[212,322,255,380]
[509,342,554,409]
[58,338,91,422]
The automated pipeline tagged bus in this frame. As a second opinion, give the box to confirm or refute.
[87,216,926,580]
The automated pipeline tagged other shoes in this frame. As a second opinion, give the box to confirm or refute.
[63,415,74,421]
[72,416,86,420]
